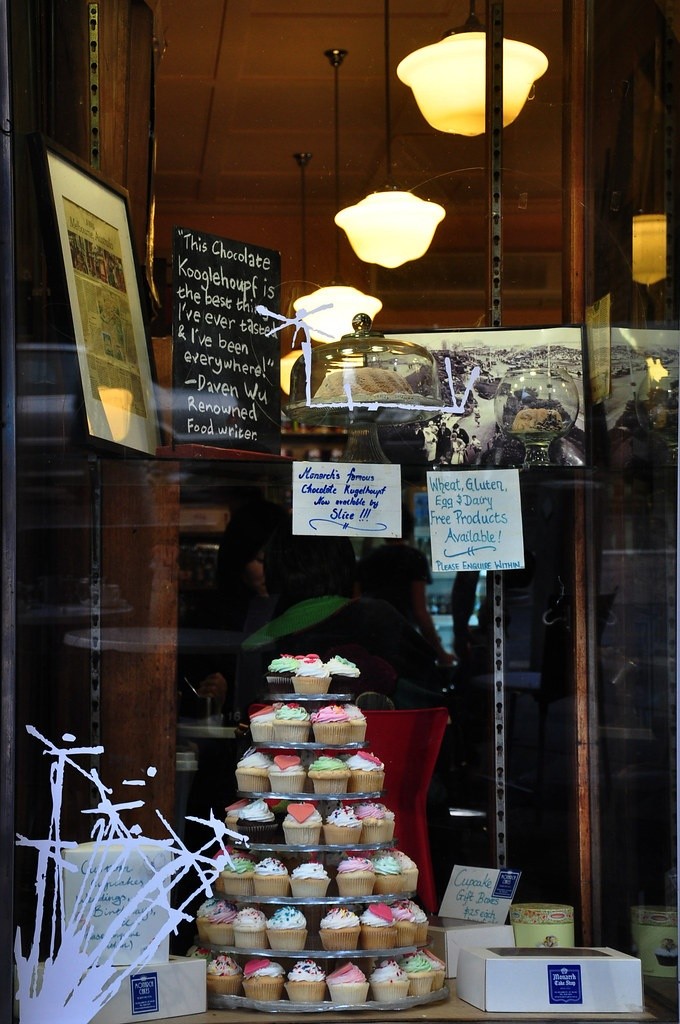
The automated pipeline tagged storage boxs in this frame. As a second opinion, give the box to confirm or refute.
[457,945,645,1013]
[423,917,512,980]
[11,958,208,1023]
[64,841,174,966]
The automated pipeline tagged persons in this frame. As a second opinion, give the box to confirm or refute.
[450,557,533,675]
[473,404,480,427]
[411,420,481,464]
[177,502,492,804]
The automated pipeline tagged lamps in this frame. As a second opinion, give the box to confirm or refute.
[333,1,448,267]
[280,153,321,395]
[293,150,384,339]
[629,214,668,291]
[394,0,549,142]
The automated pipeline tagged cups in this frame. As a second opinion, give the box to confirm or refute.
[608,658,641,693]
[194,696,224,727]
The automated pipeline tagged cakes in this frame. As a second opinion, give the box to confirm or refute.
[312,366,418,399]
[511,407,563,431]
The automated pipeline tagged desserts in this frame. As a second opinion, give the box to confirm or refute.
[185,653,447,1004]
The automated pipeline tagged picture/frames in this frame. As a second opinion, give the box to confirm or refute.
[39,126,165,460]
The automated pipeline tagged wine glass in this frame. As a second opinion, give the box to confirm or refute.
[636,367,678,466]
[495,367,579,467]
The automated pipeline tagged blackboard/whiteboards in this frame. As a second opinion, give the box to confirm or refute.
[172,223,283,455]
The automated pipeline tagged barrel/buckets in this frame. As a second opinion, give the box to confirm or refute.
[508,902,575,949]
[629,905,678,977]
